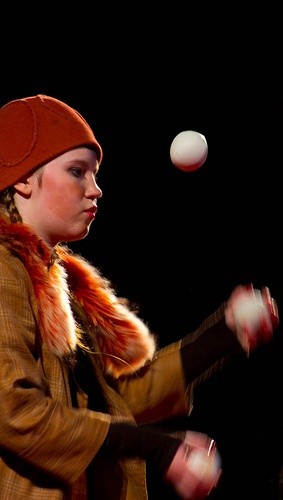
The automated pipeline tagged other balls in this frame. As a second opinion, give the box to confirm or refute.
[184,446,222,485]
[168,130,209,174]
[230,289,272,333]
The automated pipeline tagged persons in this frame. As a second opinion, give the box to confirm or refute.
[0,92,280,500]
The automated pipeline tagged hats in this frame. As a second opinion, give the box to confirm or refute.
[0,94,103,191]
